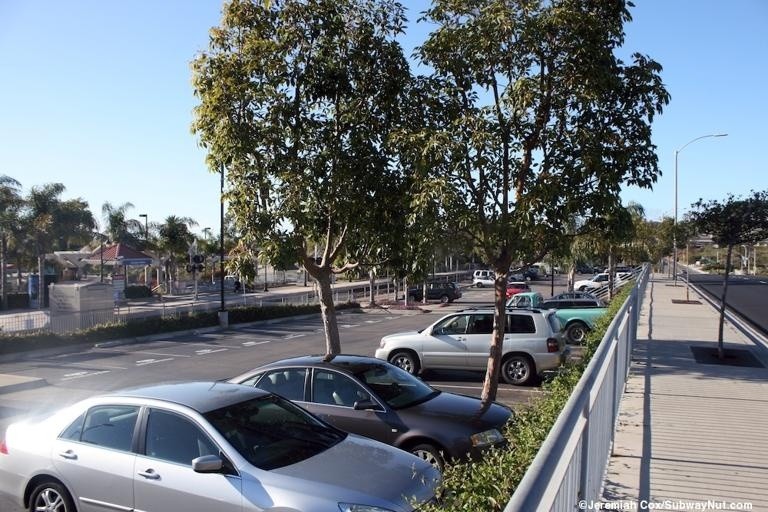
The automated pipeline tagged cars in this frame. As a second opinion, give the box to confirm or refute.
[2,379,444,512]
[375,263,640,384]
[228,354,517,474]
[673,132,728,279]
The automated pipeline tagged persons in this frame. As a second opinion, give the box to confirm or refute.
[233,278,242,293]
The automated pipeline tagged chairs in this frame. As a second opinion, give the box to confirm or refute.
[81,410,126,453]
[259,370,304,400]
[156,418,248,465]
[332,373,367,406]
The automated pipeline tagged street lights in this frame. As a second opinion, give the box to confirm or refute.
[203,227,211,284]
[139,213,150,242]
